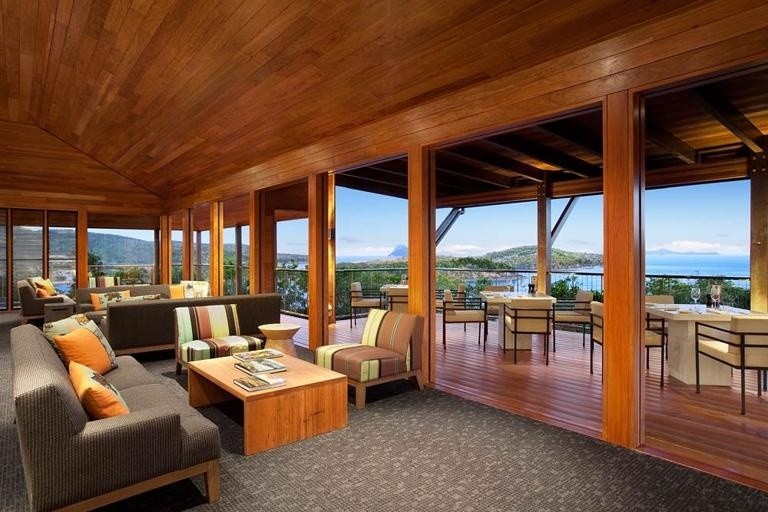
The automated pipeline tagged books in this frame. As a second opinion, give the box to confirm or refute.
[233,348,283,362]
[233,373,287,392]
[234,357,288,376]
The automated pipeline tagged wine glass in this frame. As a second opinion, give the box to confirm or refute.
[708,283,722,309]
[689,287,700,309]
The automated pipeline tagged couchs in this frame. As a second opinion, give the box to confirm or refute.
[17,276,281,356]
[9,323,220,512]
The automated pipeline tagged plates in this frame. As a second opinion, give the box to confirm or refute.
[645,304,753,318]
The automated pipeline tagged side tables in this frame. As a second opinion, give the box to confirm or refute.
[258,323,300,359]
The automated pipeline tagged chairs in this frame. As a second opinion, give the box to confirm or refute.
[172,304,263,376]
[436,285,604,384]
[644,295,768,416]
[387,287,409,313]
[349,282,387,328]
[315,307,424,410]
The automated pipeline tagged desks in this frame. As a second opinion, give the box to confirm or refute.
[379,284,408,310]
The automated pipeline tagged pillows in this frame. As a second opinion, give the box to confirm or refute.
[42,313,91,365]
[52,319,118,374]
[68,360,129,417]
